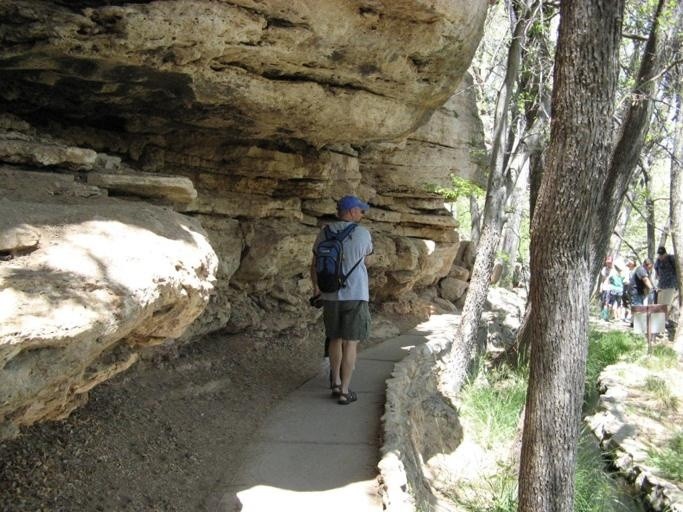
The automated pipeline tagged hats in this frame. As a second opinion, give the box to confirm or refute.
[336,196,369,209]
[658,247,665,254]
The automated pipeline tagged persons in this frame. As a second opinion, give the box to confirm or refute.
[587,245,680,326]
[311,195,374,404]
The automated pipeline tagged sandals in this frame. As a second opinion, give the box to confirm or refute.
[332,385,356,404]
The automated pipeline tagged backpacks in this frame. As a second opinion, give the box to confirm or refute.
[316,224,362,293]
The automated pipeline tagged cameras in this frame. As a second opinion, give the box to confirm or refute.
[309,294,324,309]
